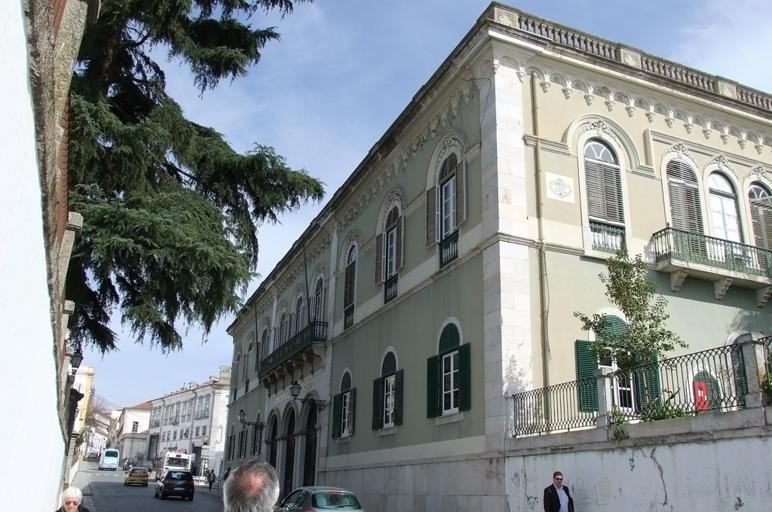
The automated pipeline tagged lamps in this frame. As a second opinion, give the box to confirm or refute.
[290,381,326,413]
[236,409,265,431]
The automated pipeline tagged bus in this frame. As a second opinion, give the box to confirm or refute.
[156,451,191,479]
[98,449,120,471]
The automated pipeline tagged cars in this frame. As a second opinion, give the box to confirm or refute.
[155,468,195,501]
[124,467,149,487]
[273,486,365,512]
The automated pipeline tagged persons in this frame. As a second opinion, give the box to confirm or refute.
[224,467,231,481]
[222,456,280,512]
[54,487,89,512]
[207,468,216,492]
[543,470,574,512]
[123,457,128,470]
